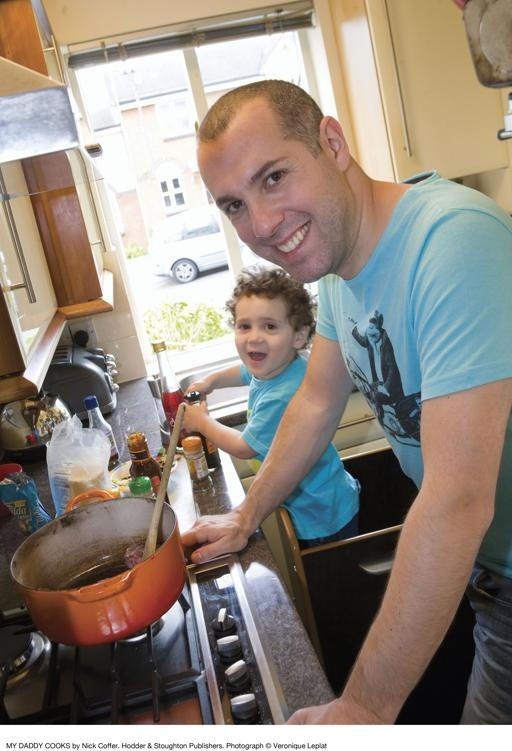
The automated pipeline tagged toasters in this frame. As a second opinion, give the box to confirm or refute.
[43,343,121,420]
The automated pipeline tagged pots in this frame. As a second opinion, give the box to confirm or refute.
[8,488,187,646]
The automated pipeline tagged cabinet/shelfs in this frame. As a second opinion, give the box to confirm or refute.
[0,0,115,404]
[313,0,512,185]
[223,393,512,725]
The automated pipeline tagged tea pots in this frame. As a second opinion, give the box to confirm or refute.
[0,388,73,462]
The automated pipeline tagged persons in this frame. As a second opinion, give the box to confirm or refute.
[350,316,404,408]
[179,262,368,674]
[179,78,512,726]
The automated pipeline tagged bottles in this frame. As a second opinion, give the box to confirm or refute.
[84,395,119,466]
[127,338,221,506]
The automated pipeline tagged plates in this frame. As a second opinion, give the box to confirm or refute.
[109,453,178,497]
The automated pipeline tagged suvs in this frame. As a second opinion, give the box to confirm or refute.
[150,203,231,286]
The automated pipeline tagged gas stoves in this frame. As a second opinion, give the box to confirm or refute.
[0,552,287,725]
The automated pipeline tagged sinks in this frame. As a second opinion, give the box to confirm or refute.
[176,357,252,413]
